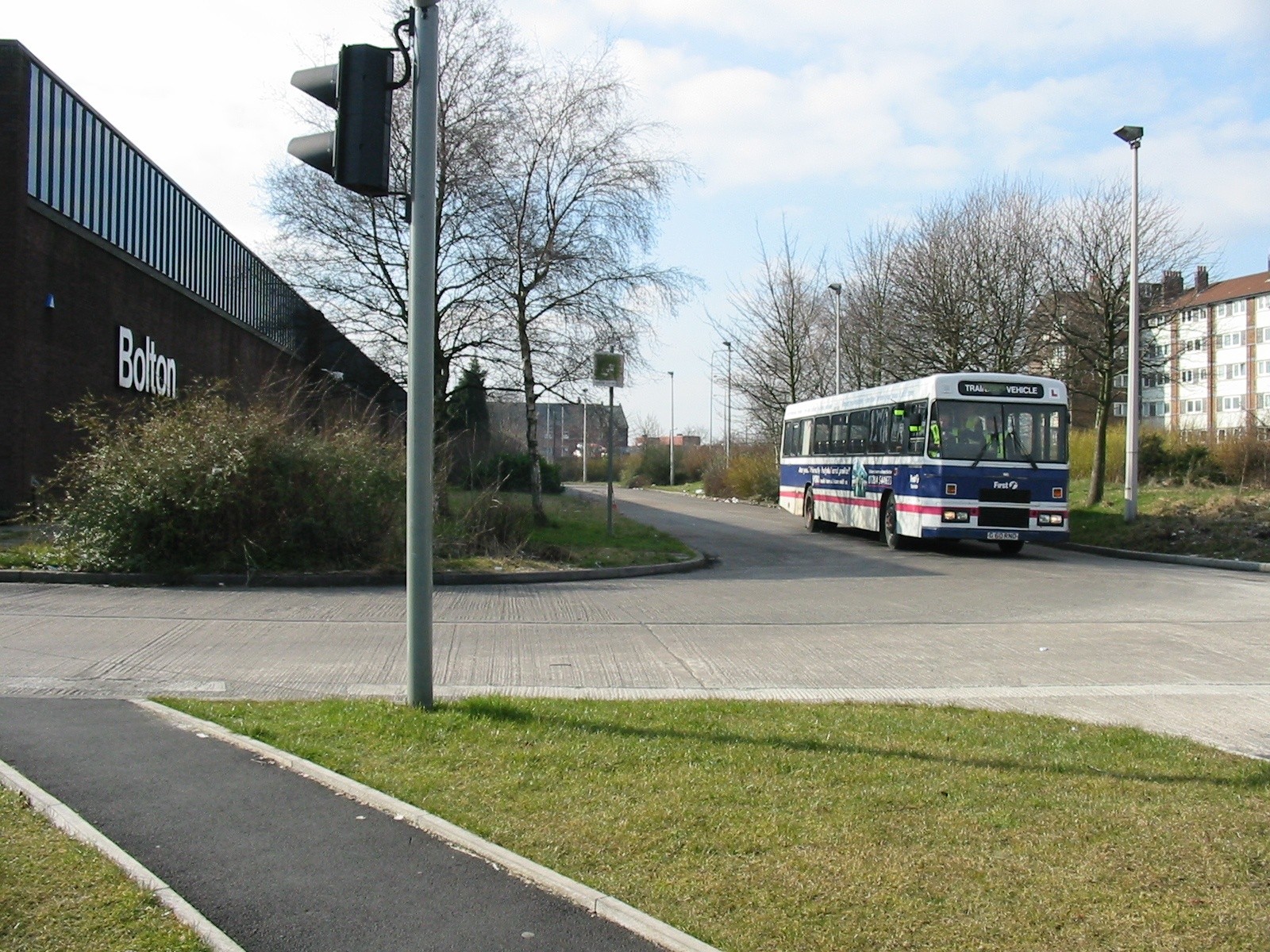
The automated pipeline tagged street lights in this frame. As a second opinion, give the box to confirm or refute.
[1112,124,1144,526]
[827,283,842,396]
[582,388,588,483]
[722,341,731,471]
[709,349,734,451]
[668,371,675,486]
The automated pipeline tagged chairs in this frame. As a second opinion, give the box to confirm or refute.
[813,439,886,453]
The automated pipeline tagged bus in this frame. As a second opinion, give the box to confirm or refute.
[777,370,1073,555]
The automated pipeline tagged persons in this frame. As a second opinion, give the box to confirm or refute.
[928,407,1014,461]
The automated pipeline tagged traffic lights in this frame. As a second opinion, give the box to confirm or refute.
[287,42,395,199]
